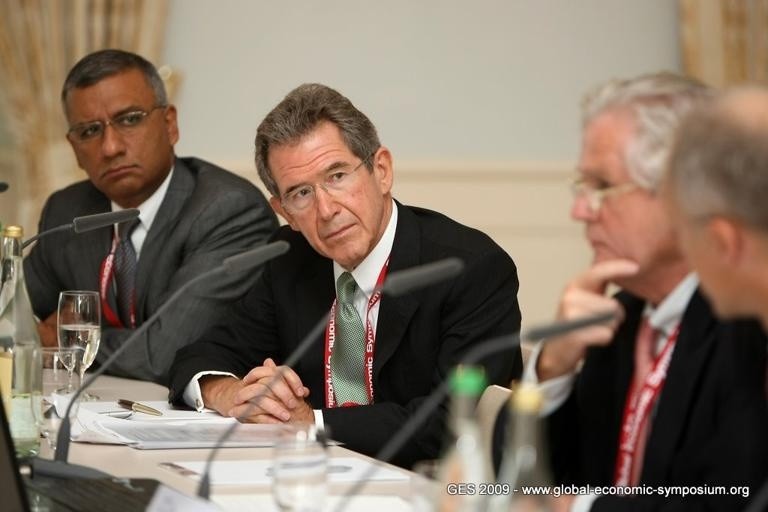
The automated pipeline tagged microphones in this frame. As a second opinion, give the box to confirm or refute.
[196,257,464,500]
[334,311,614,512]
[22,207,140,249]
[32,240,290,477]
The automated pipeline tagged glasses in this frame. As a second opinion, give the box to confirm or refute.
[69,105,166,144]
[280,152,373,215]
[571,179,640,213]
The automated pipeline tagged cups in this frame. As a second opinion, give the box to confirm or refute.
[272,435,329,512]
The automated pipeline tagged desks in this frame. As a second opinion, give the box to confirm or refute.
[2,360,498,510]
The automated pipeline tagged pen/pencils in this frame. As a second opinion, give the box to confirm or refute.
[118,399,163,416]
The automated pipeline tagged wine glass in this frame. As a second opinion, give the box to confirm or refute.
[31,347,84,464]
[56,290,102,461]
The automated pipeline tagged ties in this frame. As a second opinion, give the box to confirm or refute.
[330,270,368,407]
[108,217,141,329]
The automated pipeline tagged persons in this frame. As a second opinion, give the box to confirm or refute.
[24,48,523,482]
[519,73,768,510]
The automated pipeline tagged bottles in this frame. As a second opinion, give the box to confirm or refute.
[429,364,551,512]
[0,225,42,459]
